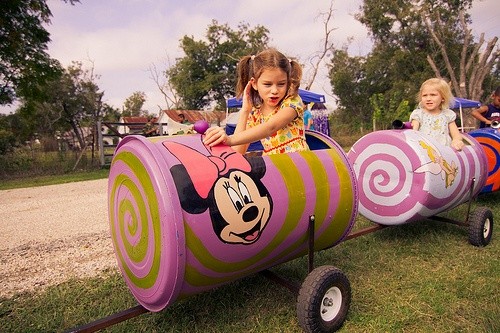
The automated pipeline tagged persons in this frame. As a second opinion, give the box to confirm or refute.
[409,78,465,151]
[471,85,500,128]
[302,98,315,130]
[142,113,160,137]
[393,120,412,131]
[176,114,194,134]
[202,50,311,154]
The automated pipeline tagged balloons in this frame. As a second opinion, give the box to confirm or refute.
[195,121,208,133]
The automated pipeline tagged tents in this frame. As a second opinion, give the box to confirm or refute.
[227,86,327,105]
[450,96,479,111]
